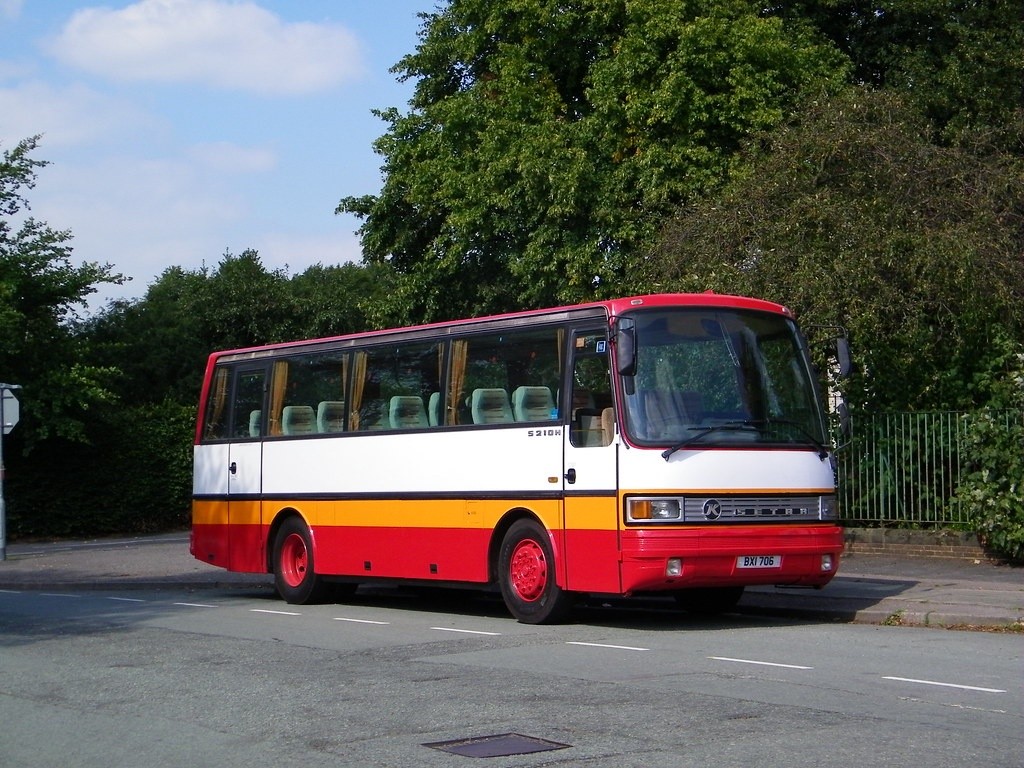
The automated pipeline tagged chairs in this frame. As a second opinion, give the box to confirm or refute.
[248,385,557,437]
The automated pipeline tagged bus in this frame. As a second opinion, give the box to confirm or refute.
[190,290,846,625]
